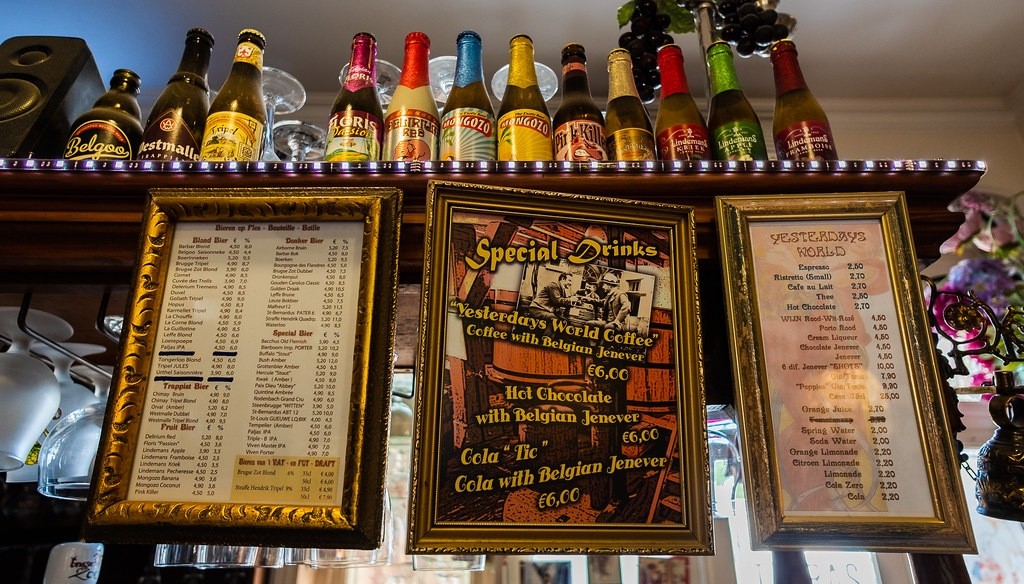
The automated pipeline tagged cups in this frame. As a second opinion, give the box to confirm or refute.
[42,542,104,584]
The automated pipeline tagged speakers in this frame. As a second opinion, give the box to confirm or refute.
[0,35,107,158]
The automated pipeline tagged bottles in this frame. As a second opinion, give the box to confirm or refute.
[199,29,267,161]
[383,32,439,161]
[656,44,707,161]
[551,42,607,161]
[772,40,840,162]
[706,40,768,160]
[441,30,496,160]
[603,47,654,160]
[497,34,551,162]
[63,68,144,160]
[324,32,381,161]
[137,28,214,163]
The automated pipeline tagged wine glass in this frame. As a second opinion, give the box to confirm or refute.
[252,55,556,160]
[155,381,741,571]
[0,307,127,507]
